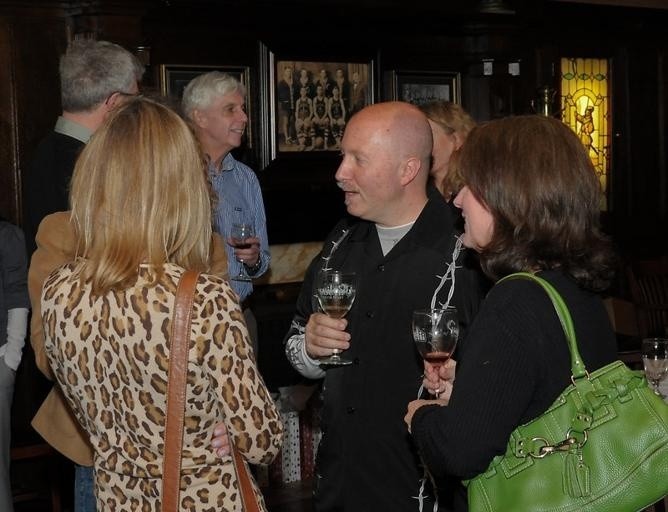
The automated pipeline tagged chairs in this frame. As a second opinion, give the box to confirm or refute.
[624,258,668,341]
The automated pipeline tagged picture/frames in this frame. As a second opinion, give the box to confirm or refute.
[270,47,381,160]
[392,69,462,106]
[160,66,251,155]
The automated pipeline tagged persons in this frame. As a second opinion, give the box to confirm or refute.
[280,99,498,510]
[404,89,445,106]
[26,40,145,511]
[420,99,477,214]
[38,92,285,511]
[181,70,273,366]
[403,115,627,512]
[0,218,31,509]
[278,66,366,151]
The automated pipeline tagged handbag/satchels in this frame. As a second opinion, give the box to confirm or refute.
[460,272,667,512]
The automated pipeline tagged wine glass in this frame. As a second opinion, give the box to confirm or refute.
[231,223,254,282]
[641,338,668,399]
[316,270,357,366]
[411,308,460,403]
